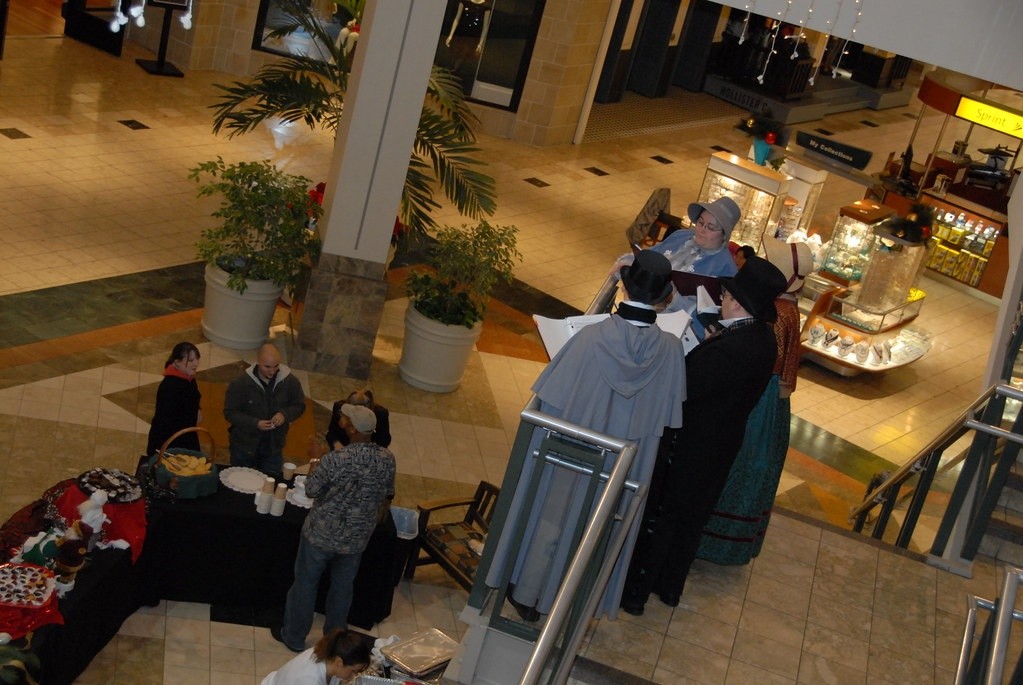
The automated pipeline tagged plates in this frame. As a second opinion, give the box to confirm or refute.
[219,466,268,494]
[286,487,315,509]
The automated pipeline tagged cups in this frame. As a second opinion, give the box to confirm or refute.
[952,141,968,157]
[254,477,288,517]
[284,463,296,480]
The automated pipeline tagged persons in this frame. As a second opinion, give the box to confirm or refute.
[445,0,491,93]
[308,0,366,65]
[270,403,395,654]
[619,257,787,616]
[223,347,306,479]
[694,232,815,566]
[484,250,686,623]
[146,341,202,456]
[733,246,756,269]
[325,364,392,451]
[641,198,741,356]
[258,627,371,685]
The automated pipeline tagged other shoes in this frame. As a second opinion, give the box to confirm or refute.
[507,589,540,622]
[271,622,305,652]
[620,586,681,616]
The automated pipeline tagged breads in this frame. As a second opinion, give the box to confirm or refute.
[164,453,212,476]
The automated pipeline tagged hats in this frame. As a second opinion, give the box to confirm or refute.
[340,403,377,434]
[687,196,741,243]
[619,250,674,305]
[717,256,788,321]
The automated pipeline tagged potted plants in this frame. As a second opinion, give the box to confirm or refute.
[734,108,791,165]
[187,156,326,349]
[389,219,523,394]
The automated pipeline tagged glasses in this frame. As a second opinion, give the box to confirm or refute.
[719,293,733,301]
[696,217,722,233]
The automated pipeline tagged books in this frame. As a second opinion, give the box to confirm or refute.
[631,242,723,307]
[529,312,612,362]
[696,285,726,333]
[614,303,700,358]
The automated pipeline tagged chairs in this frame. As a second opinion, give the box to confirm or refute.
[403,480,500,596]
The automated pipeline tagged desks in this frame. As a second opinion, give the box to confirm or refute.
[0,456,421,685]
